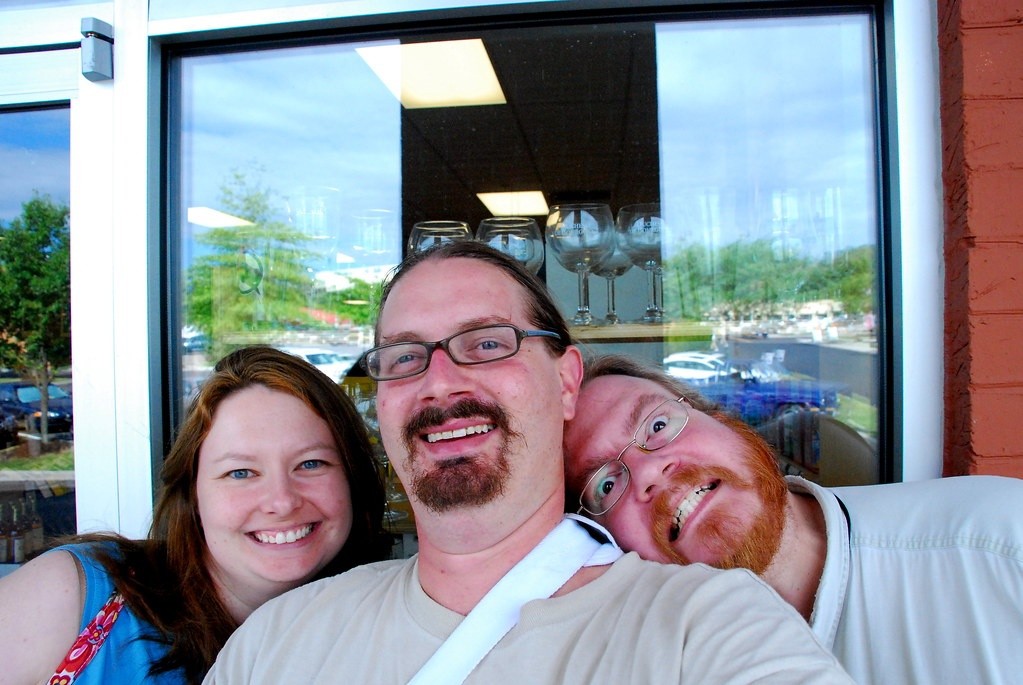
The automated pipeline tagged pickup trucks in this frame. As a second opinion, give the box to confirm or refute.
[683,357,838,424]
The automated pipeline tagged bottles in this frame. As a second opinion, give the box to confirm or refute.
[0,495,45,564]
[747,347,789,382]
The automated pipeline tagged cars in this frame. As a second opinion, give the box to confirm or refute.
[0,380,74,451]
[277,345,357,384]
[662,351,752,382]
[179,325,206,350]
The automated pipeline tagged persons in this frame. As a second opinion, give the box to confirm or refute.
[201,240,851,685]
[563,354,1023,685]
[0,346,385,685]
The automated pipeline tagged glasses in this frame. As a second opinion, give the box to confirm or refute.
[360,322,562,381]
[577,395,695,518]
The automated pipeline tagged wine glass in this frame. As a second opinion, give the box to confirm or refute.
[341,383,409,522]
[689,186,859,338]
[407,204,678,327]
[236,184,396,334]
